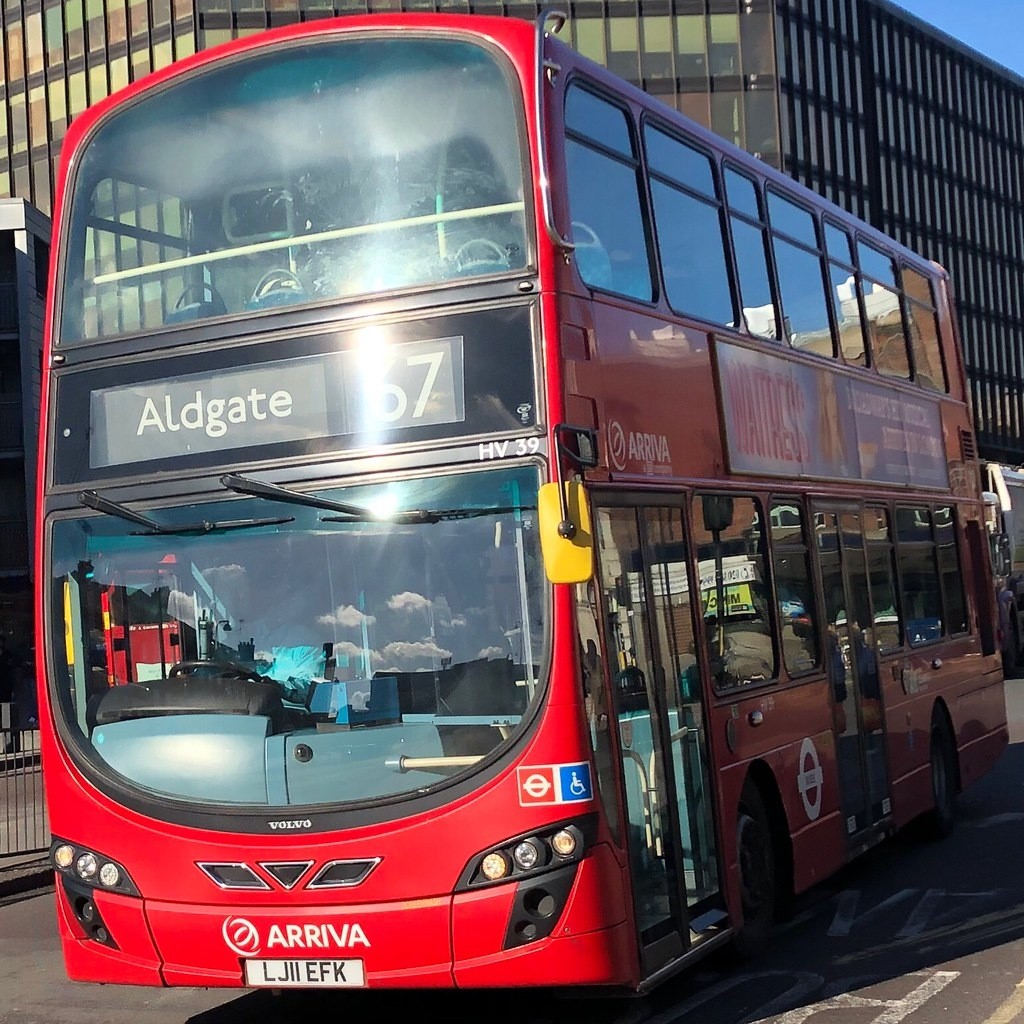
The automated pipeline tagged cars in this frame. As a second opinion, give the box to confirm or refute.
[907,616,943,646]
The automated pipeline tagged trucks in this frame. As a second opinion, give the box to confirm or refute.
[979,462,1023,680]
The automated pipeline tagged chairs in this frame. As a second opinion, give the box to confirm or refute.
[162,284,230,328]
[245,269,311,315]
[437,239,512,282]
[573,222,614,290]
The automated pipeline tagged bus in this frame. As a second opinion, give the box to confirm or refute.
[32,10,1012,1000]
[101,569,184,690]
